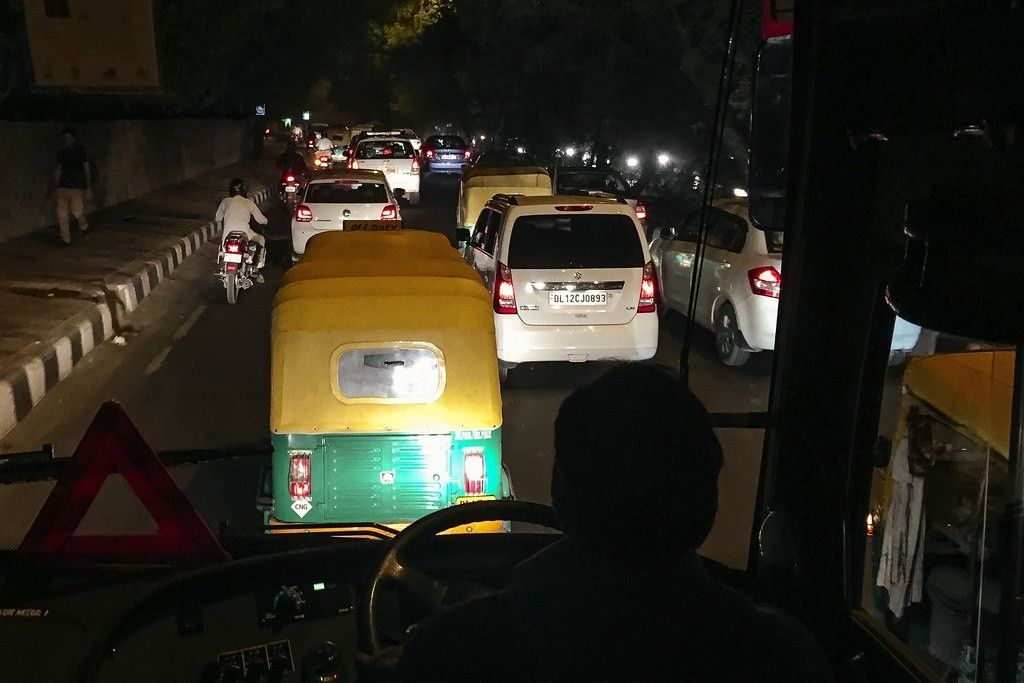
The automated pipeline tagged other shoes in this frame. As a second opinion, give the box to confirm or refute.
[57,242,70,250]
[82,224,93,234]
[300,189,305,195]
[250,271,260,278]
[280,196,286,202]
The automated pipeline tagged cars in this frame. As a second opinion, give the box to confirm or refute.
[418,135,471,176]
[663,195,923,367]
[279,117,741,250]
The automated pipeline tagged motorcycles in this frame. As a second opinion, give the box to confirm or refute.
[256,227,517,543]
[214,216,269,306]
[456,163,554,262]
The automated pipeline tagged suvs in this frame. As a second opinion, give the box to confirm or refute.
[291,168,406,265]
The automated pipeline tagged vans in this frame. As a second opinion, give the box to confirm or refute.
[454,191,663,383]
[344,130,423,206]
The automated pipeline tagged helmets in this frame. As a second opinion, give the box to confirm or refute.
[321,131,328,138]
[287,142,296,153]
[230,179,248,198]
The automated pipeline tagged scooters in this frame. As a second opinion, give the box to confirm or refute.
[279,157,307,210]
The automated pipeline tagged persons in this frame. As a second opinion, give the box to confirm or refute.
[214,176,268,277]
[274,139,309,199]
[54,125,94,248]
[353,359,837,682]
[316,132,334,150]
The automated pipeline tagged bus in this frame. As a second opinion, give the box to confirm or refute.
[743,1,1024,680]
